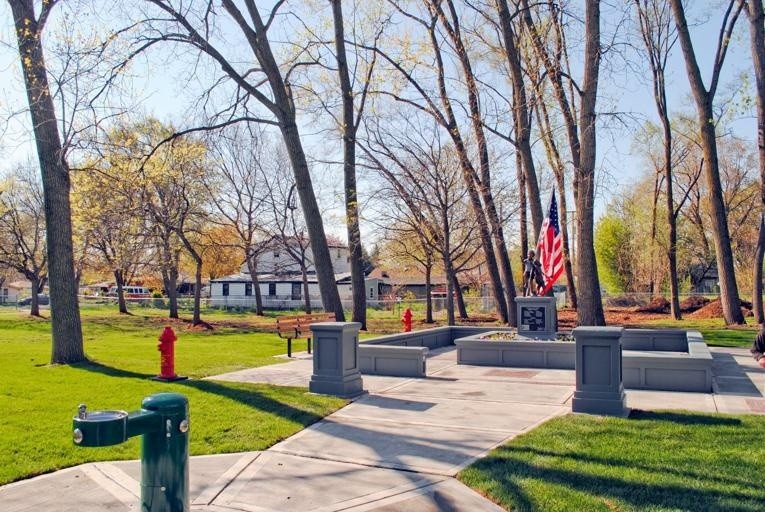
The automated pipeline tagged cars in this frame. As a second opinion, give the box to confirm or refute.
[18,292,49,306]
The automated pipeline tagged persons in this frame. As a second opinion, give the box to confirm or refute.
[750,328,765,369]
[522,250,537,297]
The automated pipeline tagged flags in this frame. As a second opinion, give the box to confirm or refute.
[533,186,565,297]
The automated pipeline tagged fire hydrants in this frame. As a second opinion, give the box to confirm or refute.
[401,308,413,332]
[155,327,178,380]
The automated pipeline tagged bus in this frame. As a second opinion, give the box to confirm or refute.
[110,285,152,303]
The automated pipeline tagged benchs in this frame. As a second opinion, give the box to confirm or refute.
[277,312,337,358]
